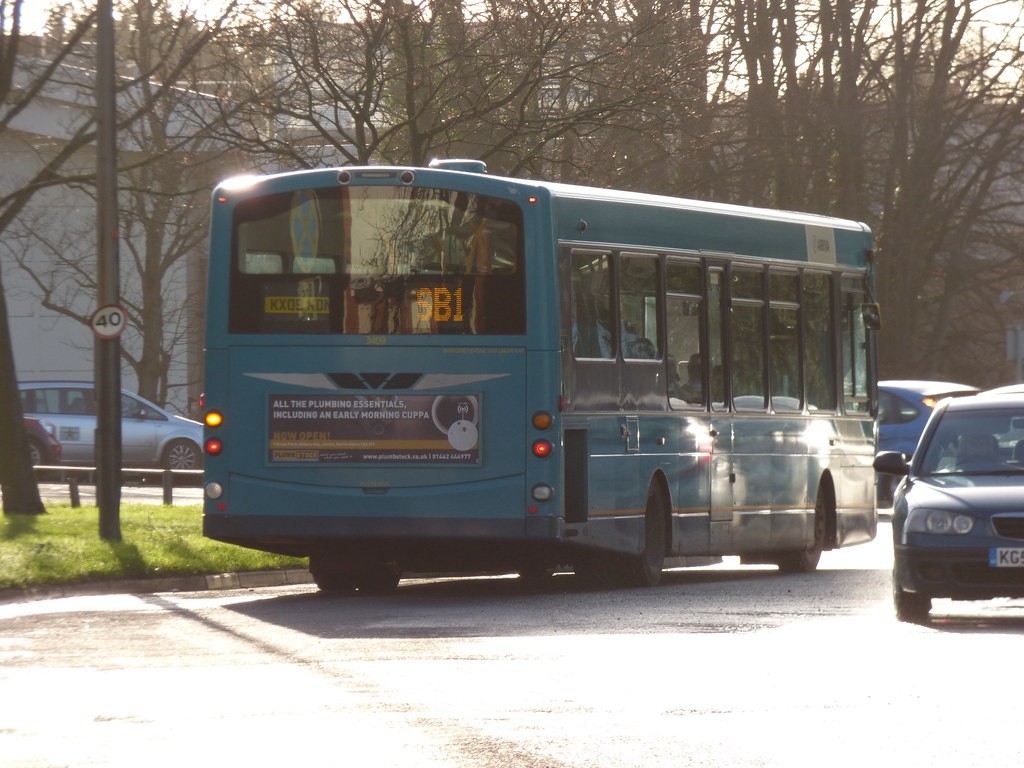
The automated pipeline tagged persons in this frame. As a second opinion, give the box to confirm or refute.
[668,351,714,403]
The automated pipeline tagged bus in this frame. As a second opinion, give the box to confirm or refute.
[201,160,882,593]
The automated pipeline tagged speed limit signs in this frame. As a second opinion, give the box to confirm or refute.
[90,306,129,339]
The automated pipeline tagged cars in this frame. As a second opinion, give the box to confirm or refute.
[24,418,60,472]
[876,379,983,504]
[874,393,1024,619]
[19,381,206,483]
[955,383,1024,467]
